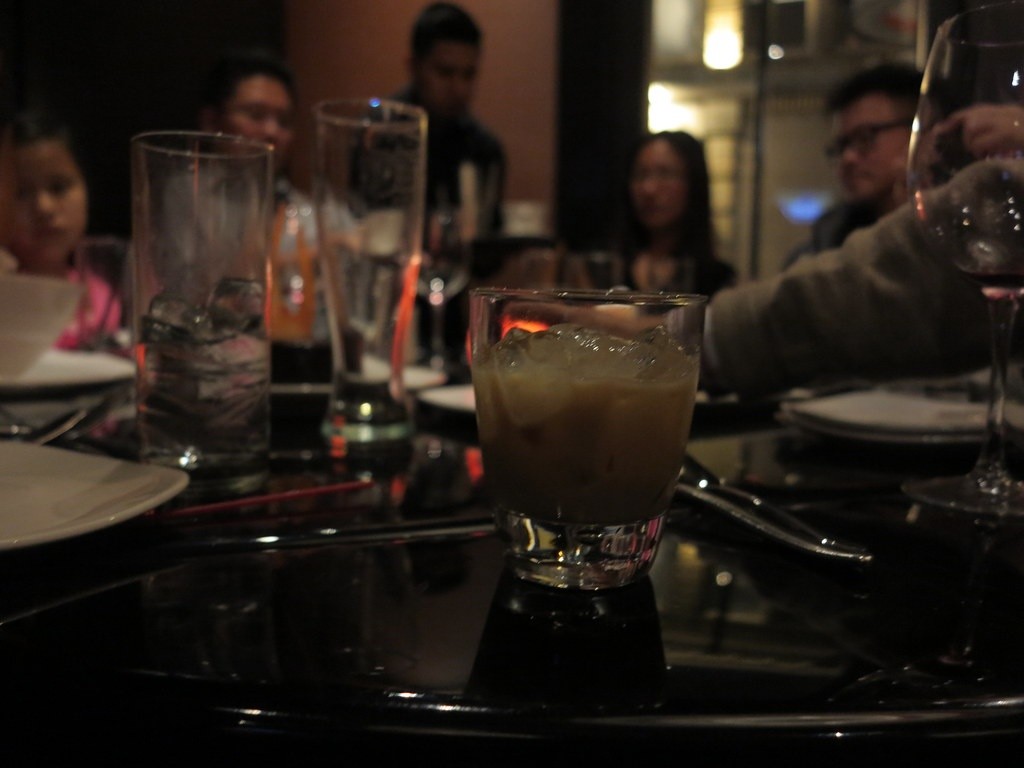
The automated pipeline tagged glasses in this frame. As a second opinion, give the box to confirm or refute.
[822,116,915,158]
[220,101,300,130]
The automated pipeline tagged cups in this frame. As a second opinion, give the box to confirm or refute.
[312,100,428,444]
[468,287,708,590]
[129,130,275,498]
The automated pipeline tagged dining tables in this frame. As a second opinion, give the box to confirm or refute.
[0,376,1024,768]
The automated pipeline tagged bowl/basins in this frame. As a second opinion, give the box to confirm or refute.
[0,273,85,383]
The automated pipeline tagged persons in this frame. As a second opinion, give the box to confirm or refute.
[782,62,976,268]
[351,0,509,348]
[124,65,394,342]
[544,132,731,310]
[508,100,1024,400]
[1,114,141,432]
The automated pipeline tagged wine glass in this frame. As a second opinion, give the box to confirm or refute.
[902,1,1024,522]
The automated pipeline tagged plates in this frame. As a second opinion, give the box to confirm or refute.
[1,346,134,386]
[417,382,476,413]
[0,438,192,551]
[776,388,1024,448]
